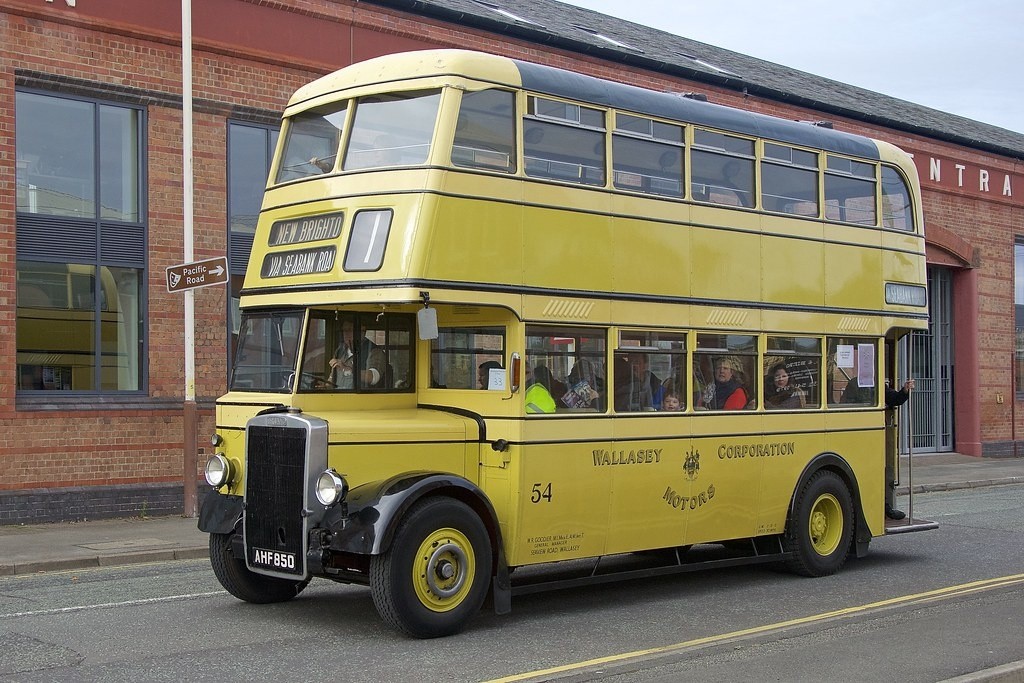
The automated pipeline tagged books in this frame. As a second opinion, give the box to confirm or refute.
[561,379,595,409]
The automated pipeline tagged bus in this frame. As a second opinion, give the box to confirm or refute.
[198,49,940,641]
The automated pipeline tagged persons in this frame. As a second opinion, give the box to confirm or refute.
[310,97,401,175]
[317,317,917,520]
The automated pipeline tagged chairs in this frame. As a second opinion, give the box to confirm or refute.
[795,386,807,408]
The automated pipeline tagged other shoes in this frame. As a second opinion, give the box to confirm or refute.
[885,503,906,520]
